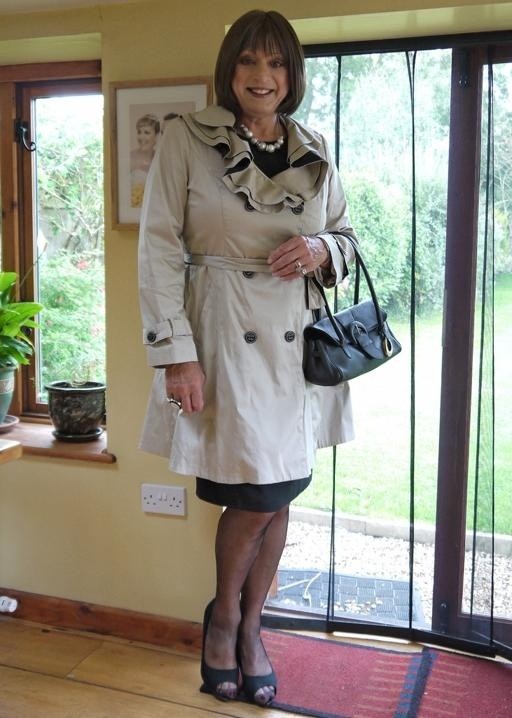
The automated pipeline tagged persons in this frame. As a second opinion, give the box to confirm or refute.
[130,114,184,208]
[138,10,360,704]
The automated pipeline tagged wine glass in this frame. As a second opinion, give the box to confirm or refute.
[295,260,307,275]
[167,398,181,409]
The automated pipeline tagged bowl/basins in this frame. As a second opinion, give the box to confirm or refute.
[240,123,284,152]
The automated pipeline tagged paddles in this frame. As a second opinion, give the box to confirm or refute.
[201,623,512,717]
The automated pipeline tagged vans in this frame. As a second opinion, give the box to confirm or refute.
[200,597,278,706]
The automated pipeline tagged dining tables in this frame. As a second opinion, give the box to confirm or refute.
[1,264,40,434]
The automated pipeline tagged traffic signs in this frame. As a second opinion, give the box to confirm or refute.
[141,482,187,518]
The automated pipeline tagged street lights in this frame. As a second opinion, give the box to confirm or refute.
[302,298,403,386]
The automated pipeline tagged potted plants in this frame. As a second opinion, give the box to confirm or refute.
[109,74,214,233]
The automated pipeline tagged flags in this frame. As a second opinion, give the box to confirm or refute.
[46,380,105,442]
